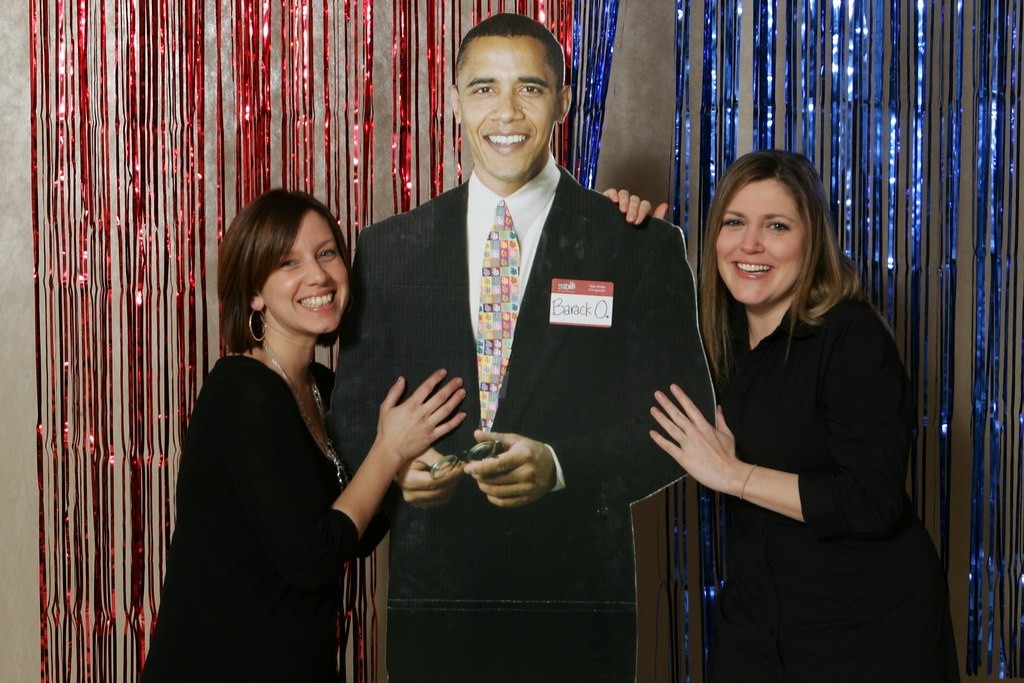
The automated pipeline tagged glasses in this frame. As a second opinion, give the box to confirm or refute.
[430,439,503,480]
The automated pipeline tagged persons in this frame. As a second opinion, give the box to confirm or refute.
[600,147,955,683]
[322,13,718,683]
[132,190,468,683]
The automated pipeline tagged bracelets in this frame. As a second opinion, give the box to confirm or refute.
[738,465,758,501]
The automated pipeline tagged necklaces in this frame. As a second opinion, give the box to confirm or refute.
[265,340,352,495]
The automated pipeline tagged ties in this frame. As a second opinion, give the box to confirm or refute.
[475,199,521,436]
